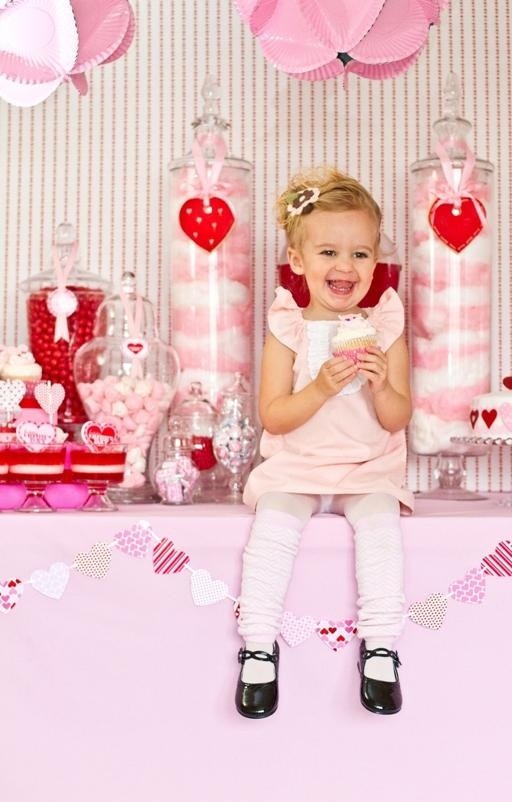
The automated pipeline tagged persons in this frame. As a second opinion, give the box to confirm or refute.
[235,164,413,719]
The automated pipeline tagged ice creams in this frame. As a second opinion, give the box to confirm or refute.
[332,315,376,373]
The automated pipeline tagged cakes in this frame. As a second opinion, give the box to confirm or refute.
[469,392,512,439]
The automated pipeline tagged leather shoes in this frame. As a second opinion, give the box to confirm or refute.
[359,640,400,712]
[237,642,278,716]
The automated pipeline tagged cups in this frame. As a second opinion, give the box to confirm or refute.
[277,230,401,307]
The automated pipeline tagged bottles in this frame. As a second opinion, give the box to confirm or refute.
[154,436,205,502]
[149,379,233,503]
[25,224,108,432]
[77,269,179,505]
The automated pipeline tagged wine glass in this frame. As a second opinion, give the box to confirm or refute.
[215,390,257,499]
[166,77,258,494]
[6,441,128,511]
[409,78,495,498]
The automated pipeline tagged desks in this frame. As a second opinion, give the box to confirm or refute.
[0,491,512,802]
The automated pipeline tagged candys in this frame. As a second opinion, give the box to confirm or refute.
[27,286,108,426]
[76,373,176,489]
[154,391,256,499]
